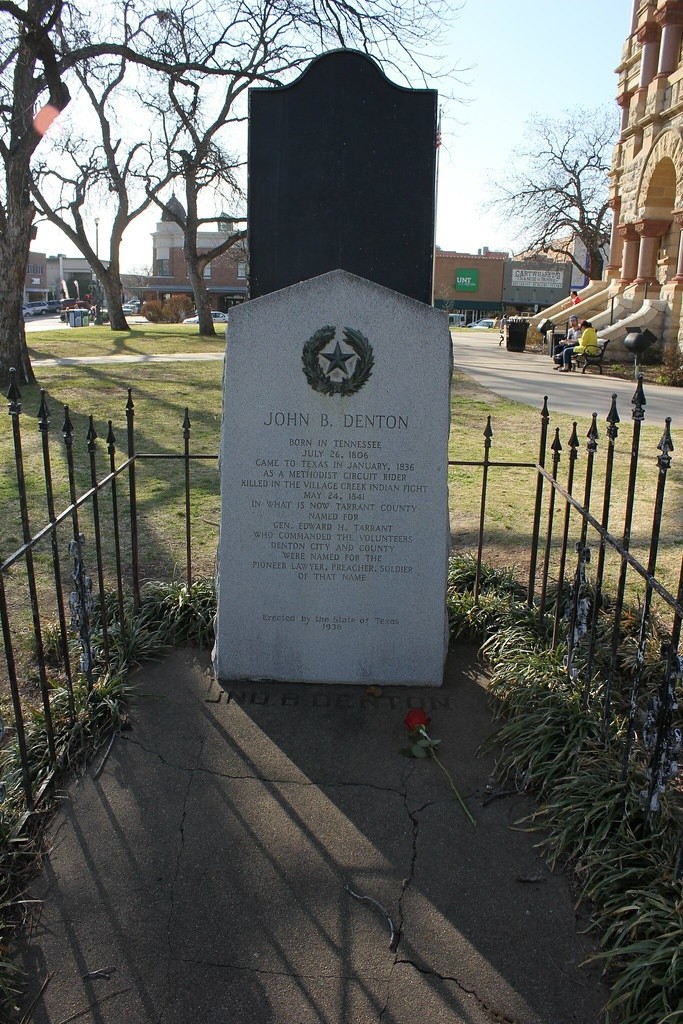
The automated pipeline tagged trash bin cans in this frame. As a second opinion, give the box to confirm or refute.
[504,318,530,353]
[69,308,89,328]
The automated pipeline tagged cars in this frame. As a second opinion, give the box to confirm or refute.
[26,302,50,315]
[182,310,229,324]
[120,299,147,316]
[20,306,34,317]
[466,318,495,328]
[47,299,76,313]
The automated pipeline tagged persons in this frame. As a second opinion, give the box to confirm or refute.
[558,319,597,372]
[554,316,582,370]
[562,291,581,310]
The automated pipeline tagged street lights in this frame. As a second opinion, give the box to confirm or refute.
[92,216,103,261]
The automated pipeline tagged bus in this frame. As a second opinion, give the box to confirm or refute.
[448,314,468,326]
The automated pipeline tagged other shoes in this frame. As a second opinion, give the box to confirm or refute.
[558,367,568,372]
[553,366,561,370]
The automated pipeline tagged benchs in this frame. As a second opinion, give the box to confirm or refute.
[571,337,610,374]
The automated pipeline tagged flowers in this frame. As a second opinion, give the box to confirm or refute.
[396,707,476,826]
